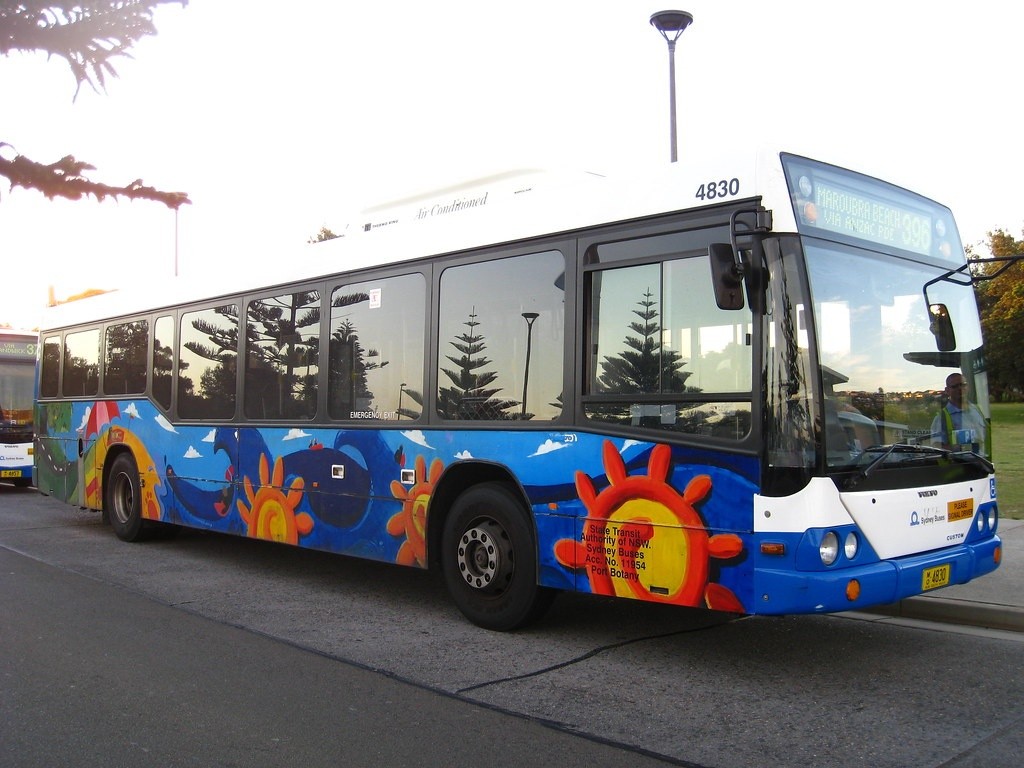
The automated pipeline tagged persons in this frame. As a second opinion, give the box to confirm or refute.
[930,374,985,454]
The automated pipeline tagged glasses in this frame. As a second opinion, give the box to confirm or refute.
[948,382,968,389]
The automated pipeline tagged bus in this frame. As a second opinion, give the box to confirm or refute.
[31,138,1023,634]
[0,329,39,488]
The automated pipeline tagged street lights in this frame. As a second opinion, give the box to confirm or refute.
[521,312,539,416]
[649,10,694,162]
